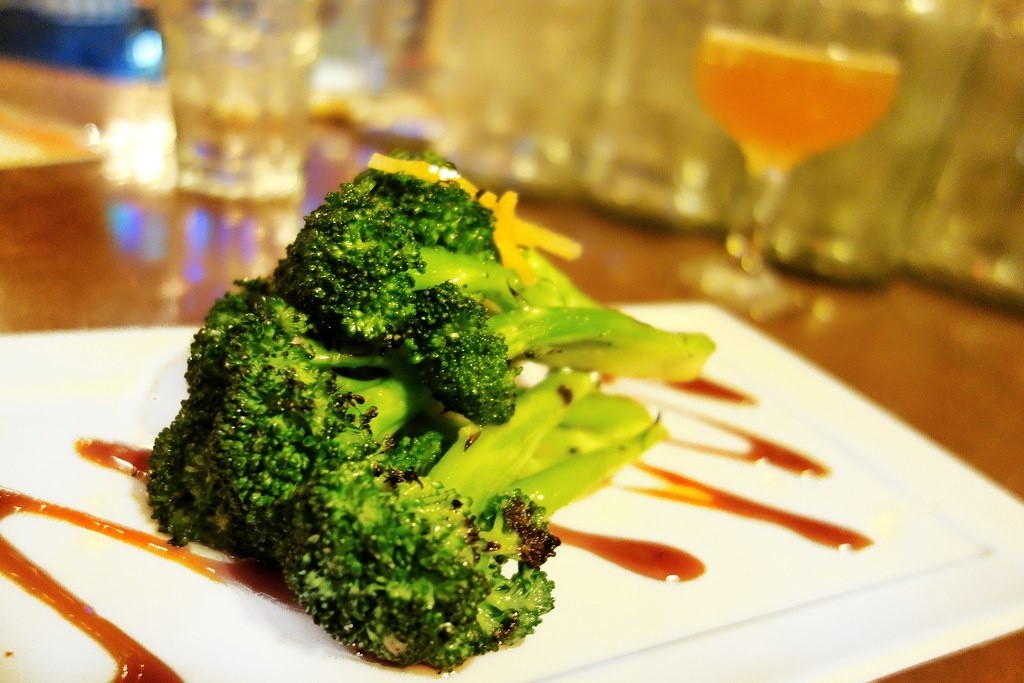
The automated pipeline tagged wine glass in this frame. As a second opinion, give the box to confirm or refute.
[681,1,905,313]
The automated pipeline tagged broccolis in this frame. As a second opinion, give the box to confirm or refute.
[147,149,713,671]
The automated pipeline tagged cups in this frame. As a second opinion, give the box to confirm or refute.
[157,1,307,205]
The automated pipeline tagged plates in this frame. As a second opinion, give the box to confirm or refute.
[1,297,1024,683]
